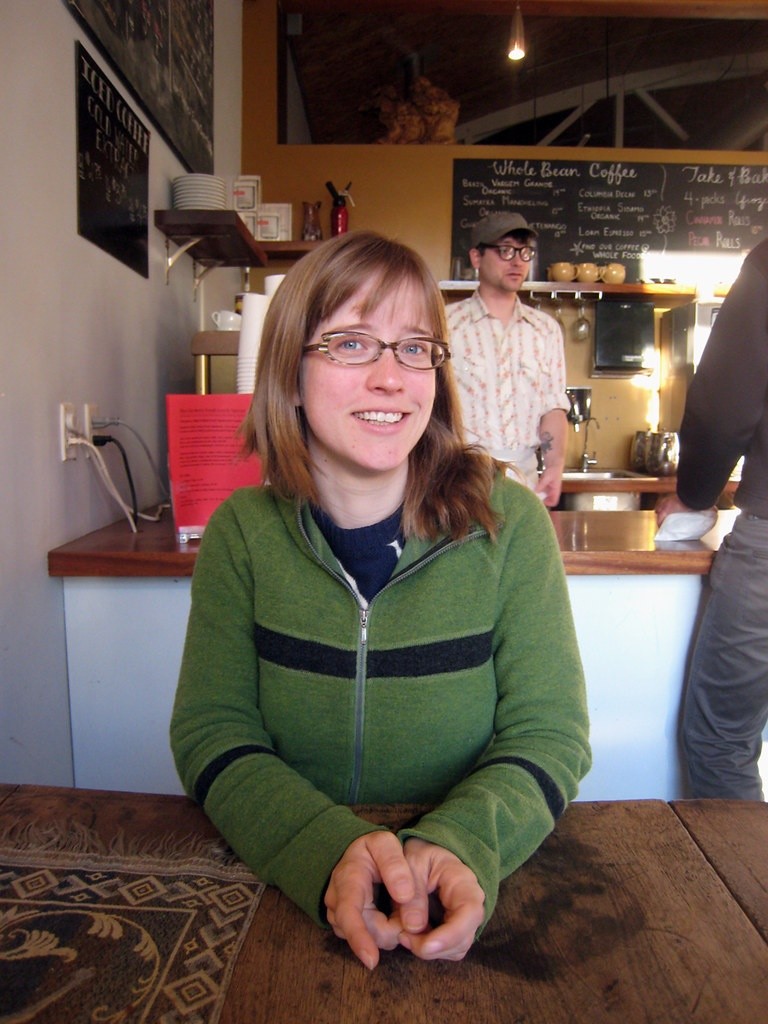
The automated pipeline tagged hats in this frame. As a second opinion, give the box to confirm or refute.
[472,211,538,249]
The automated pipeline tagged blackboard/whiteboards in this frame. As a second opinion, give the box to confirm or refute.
[450,157,768,284]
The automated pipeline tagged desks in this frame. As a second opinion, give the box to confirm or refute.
[0,782,768,1024]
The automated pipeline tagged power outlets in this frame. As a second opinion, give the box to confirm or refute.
[85,403,103,458]
[61,404,78,462]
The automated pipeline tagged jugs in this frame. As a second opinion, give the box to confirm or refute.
[301,200,323,240]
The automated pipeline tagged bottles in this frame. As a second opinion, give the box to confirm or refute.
[327,179,353,236]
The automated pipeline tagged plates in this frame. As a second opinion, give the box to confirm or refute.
[172,173,226,210]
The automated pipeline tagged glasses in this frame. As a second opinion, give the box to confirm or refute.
[478,243,536,262]
[302,330,451,370]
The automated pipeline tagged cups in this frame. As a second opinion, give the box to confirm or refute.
[644,426,680,477]
[213,312,241,331]
[547,262,580,282]
[599,262,626,283]
[578,262,605,283]
[631,428,655,470]
[451,257,466,280]
[236,273,287,394]
[524,239,539,281]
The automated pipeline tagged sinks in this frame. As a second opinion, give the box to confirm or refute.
[536,469,659,482]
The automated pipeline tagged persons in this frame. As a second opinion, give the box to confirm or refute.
[166,228,592,971]
[653,236,768,800]
[443,211,571,511]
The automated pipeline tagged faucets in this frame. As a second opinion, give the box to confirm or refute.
[579,416,601,473]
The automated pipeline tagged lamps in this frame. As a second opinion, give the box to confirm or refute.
[508,0,525,61]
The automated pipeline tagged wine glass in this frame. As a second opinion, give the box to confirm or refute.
[536,298,590,340]
